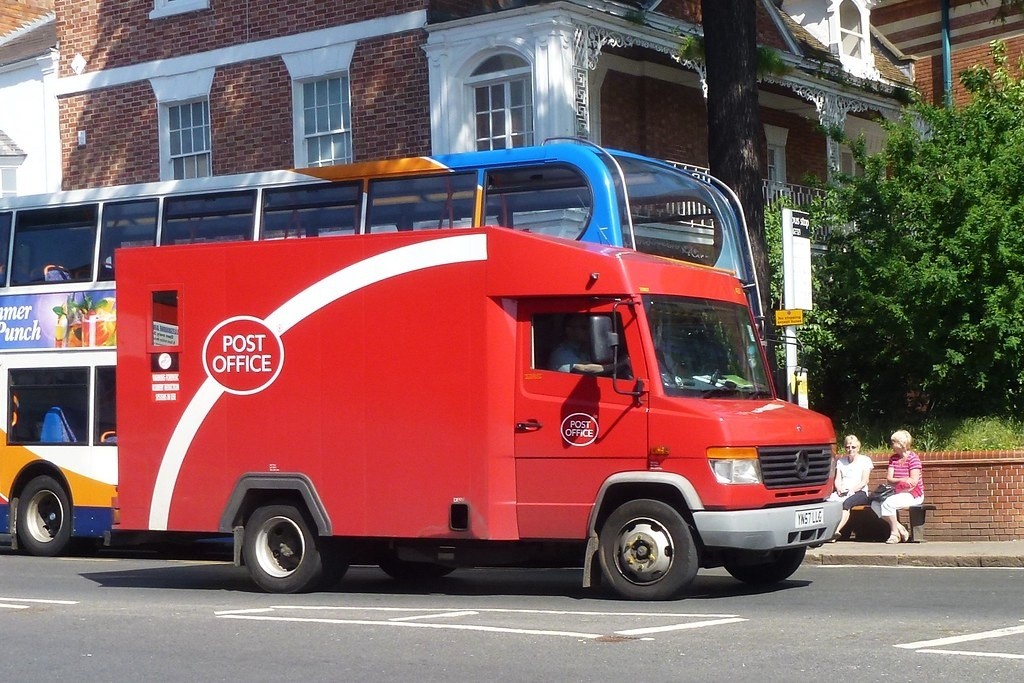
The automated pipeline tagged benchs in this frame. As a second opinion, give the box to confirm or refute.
[850,504,936,541]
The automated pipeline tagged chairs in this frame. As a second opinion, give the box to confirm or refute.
[42,262,114,282]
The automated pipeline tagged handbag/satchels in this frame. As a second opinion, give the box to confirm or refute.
[868,483,895,502]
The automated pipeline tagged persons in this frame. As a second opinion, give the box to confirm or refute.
[871,429,924,544]
[825,434,875,536]
[548,314,728,378]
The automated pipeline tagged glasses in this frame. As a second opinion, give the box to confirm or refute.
[569,325,590,330]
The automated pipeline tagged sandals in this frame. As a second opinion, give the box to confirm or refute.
[900,527,910,542]
[885,534,901,544]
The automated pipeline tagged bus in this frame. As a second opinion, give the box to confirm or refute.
[1,136,809,558]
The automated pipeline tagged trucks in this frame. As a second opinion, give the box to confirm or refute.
[108,223,838,601]
